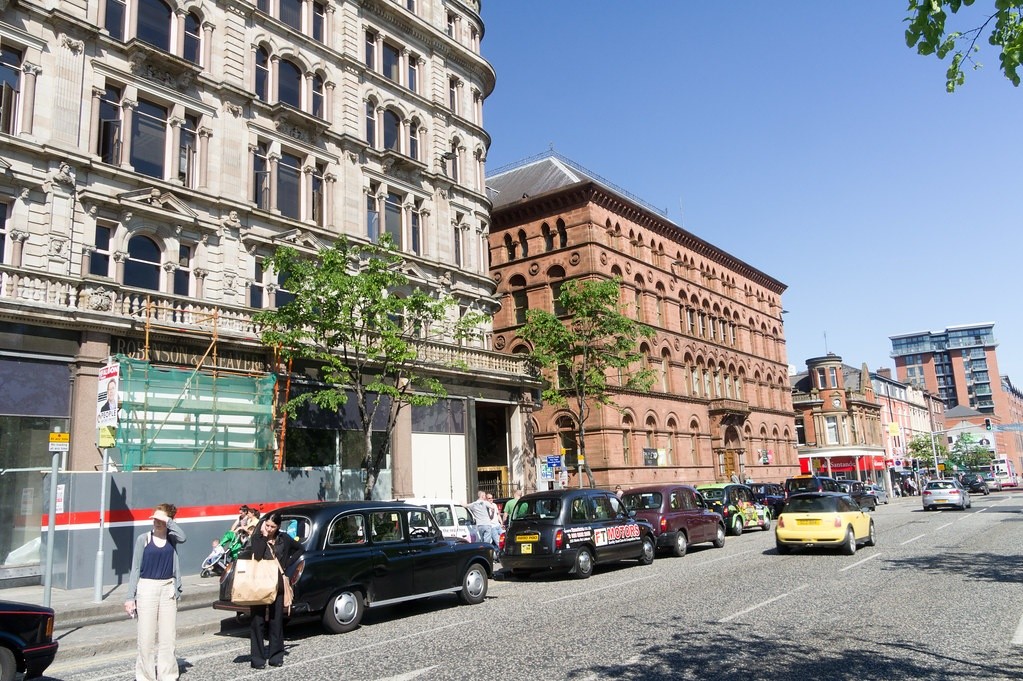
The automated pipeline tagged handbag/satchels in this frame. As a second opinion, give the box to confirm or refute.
[219,540,295,609]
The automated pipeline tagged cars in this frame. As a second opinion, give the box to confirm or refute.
[0,598,58,681]
[945,473,1002,495]
[695,483,772,535]
[375,497,482,545]
[495,488,656,578]
[923,479,972,511]
[211,500,497,635]
[774,491,875,555]
[745,475,890,519]
[616,485,727,557]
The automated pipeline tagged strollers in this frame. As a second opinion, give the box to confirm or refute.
[199,529,239,578]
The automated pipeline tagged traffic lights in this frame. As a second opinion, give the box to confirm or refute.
[984,418,993,430]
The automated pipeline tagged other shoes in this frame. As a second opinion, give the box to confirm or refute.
[275,663,281,667]
[257,665,265,670]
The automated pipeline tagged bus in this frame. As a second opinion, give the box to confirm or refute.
[990,459,1019,488]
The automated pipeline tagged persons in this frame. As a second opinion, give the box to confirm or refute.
[207,539,225,565]
[251,512,305,669]
[904,476,917,496]
[959,473,963,483]
[101,380,116,412]
[921,476,928,487]
[544,502,557,512]
[746,476,752,483]
[461,489,494,544]
[503,490,528,524]
[230,504,260,543]
[124,502,187,681]
[574,500,580,511]
[867,479,872,484]
[486,494,505,547]
[223,210,243,230]
[615,485,623,497]
[730,471,739,483]
[442,271,452,286]
[694,484,697,490]
[53,160,76,185]
